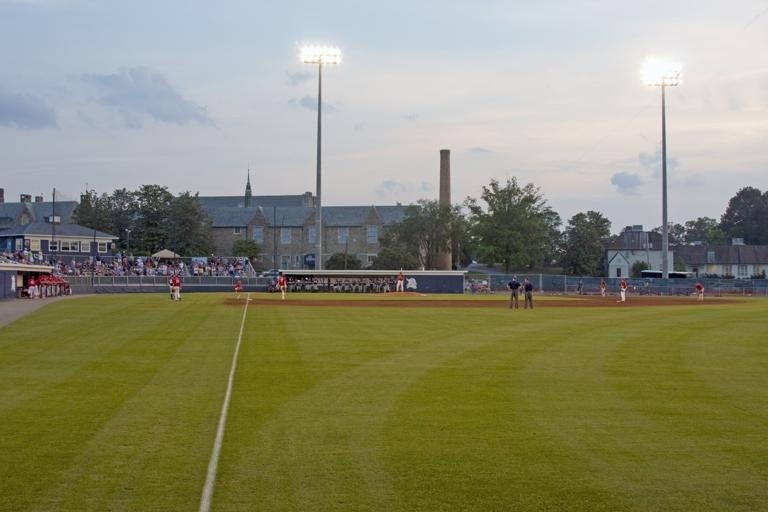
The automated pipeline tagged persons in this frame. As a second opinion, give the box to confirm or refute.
[695,283,705,302]
[1,247,251,278]
[278,272,288,301]
[169,274,176,300]
[172,274,182,300]
[395,272,405,292]
[507,275,521,309]
[27,278,72,299]
[234,279,242,291]
[600,278,606,297]
[620,278,627,302]
[521,277,533,309]
[266,275,393,294]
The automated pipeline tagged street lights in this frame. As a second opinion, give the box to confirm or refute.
[301,47,341,270]
[125,229,131,256]
[642,60,681,278]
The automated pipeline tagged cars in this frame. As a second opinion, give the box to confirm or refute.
[263,269,279,277]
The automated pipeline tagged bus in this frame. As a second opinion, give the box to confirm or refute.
[641,270,696,278]
